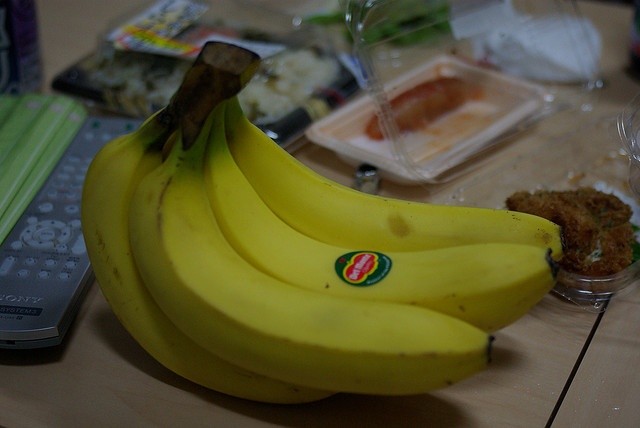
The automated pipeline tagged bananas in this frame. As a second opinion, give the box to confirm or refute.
[79,38,570,406]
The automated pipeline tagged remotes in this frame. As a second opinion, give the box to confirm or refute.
[1,114,144,362]
